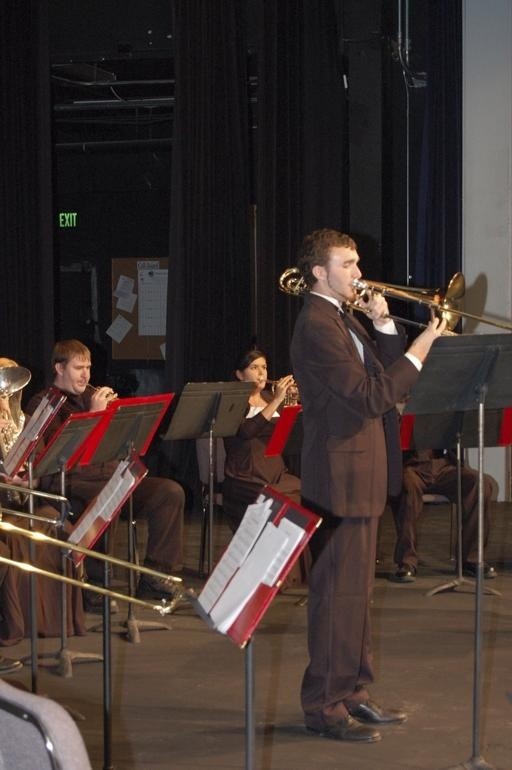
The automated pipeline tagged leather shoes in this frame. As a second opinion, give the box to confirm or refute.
[306,713,382,743]
[348,699,407,724]
[396,564,417,582]
[138,576,173,599]
[458,560,498,578]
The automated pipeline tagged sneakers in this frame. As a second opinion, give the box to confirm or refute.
[84,590,119,614]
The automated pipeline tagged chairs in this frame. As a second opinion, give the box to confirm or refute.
[0,676,92,770]
[195,436,227,580]
[376,493,455,565]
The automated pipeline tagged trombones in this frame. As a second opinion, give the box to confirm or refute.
[278,266,512,337]
[1,523,184,616]
[1,483,69,525]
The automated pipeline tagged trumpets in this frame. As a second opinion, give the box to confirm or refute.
[257,380,299,405]
[85,384,120,402]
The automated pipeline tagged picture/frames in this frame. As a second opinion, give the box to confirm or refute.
[103,247,169,369]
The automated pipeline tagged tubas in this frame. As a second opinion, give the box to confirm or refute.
[0,366,33,459]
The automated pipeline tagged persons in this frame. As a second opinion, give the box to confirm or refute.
[0,412,88,647]
[291,229,447,746]
[220,349,314,593]
[386,448,498,584]
[19,339,199,616]
